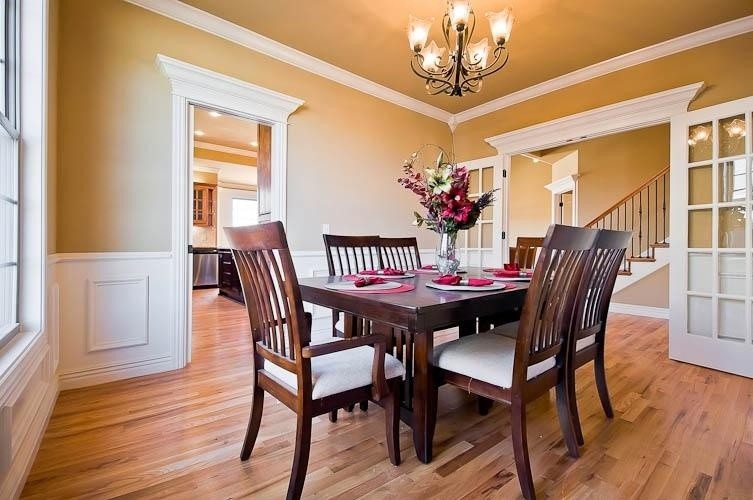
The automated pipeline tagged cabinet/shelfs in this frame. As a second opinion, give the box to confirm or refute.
[217,253,244,303]
[687,255,752,341]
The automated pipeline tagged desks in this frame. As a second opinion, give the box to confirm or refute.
[277,264,607,465]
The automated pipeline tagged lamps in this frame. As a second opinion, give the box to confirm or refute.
[405,0,517,98]
[687,117,746,157]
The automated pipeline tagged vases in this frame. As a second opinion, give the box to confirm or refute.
[724,232,734,248]
[433,225,460,278]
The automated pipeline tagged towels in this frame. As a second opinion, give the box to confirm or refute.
[353,262,534,287]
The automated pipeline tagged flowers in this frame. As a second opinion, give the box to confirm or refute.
[394,141,502,260]
[718,207,746,236]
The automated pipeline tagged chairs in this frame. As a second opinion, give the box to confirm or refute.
[322,230,402,422]
[376,233,432,379]
[420,223,597,500]
[478,228,636,447]
[222,221,405,500]
[478,235,544,335]
